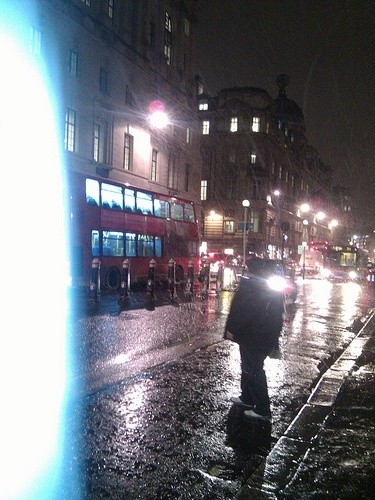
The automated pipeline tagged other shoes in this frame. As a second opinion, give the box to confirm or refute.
[244,410,269,421]
[232,396,255,408]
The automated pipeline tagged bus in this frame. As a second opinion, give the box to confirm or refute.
[323,245,368,282]
[73,167,201,294]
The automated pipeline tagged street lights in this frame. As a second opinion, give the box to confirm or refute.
[240,199,251,266]
[299,204,309,279]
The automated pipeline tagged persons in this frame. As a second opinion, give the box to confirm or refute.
[223,258,285,421]
[289,266,295,282]
